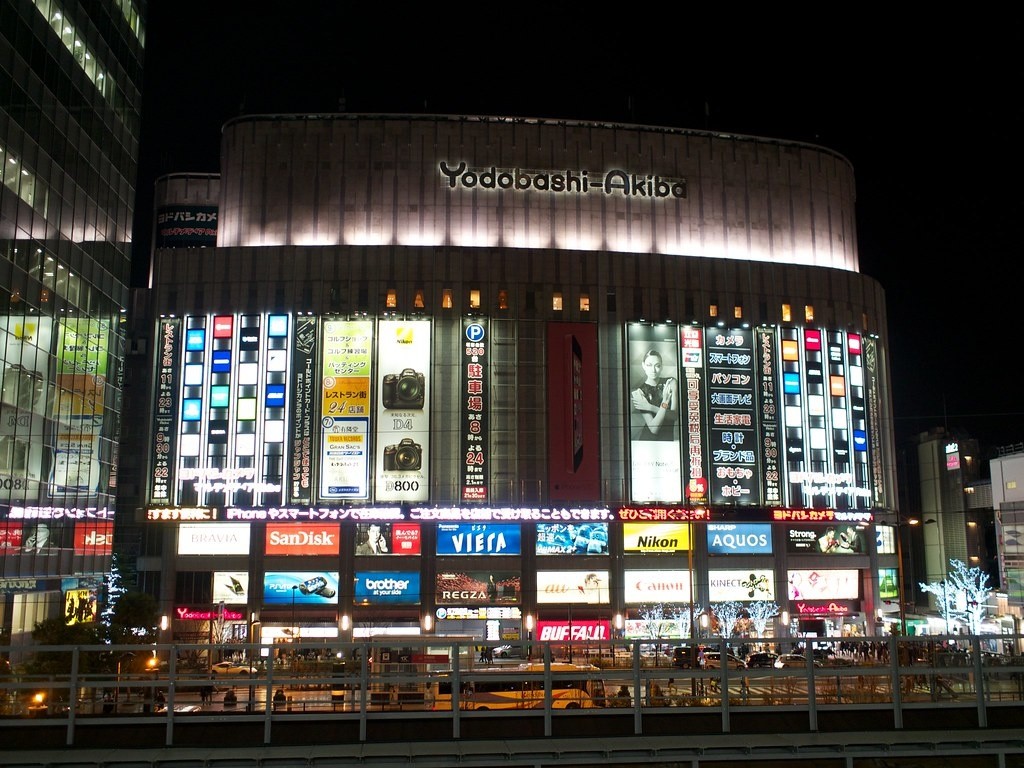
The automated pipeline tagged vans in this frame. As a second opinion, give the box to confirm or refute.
[674,647,719,670]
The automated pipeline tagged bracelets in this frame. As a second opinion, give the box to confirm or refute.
[660,402,670,412]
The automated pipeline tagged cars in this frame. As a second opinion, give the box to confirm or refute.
[211,659,259,677]
[745,652,780,668]
[494,644,521,659]
[702,652,749,669]
[774,654,824,669]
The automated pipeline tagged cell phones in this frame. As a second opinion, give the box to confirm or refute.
[564,333,583,473]
[315,587,335,598]
[299,577,328,595]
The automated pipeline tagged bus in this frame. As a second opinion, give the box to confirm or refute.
[428,663,606,711]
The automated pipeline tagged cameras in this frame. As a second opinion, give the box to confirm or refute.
[383,368,425,410]
[383,438,422,472]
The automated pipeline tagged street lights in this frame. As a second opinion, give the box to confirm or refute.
[876,509,938,696]
[686,494,707,698]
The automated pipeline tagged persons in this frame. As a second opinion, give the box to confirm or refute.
[668,677,677,696]
[199,685,212,705]
[630,350,680,441]
[22,522,59,555]
[487,574,497,602]
[765,643,770,653]
[156,690,164,709]
[844,638,993,669]
[479,646,494,664]
[355,523,388,555]
[740,676,751,694]
[741,643,748,659]
[817,525,862,554]
[775,643,782,654]
